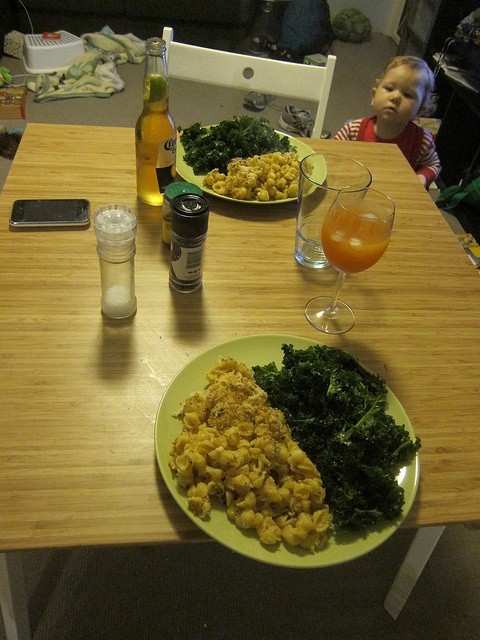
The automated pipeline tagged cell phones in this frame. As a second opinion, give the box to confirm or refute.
[9,197,93,231]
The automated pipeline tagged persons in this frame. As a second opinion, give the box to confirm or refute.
[331,56,443,190]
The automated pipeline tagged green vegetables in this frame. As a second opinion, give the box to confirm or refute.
[251,339,423,531]
[177,114,297,176]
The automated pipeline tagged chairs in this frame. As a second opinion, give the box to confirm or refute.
[162,26,337,140]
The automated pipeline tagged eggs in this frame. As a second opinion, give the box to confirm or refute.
[204,151,316,199]
[169,357,335,553]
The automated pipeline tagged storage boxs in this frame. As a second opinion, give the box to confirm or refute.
[21,30,86,75]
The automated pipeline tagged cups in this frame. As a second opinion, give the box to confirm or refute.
[294,153,372,272]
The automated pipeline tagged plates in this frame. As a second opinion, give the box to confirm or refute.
[156,334,425,570]
[179,118,331,202]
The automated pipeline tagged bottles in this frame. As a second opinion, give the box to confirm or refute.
[92,208,138,321]
[134,38,175,209]
[161,180,202,247]
[168,194,210,294]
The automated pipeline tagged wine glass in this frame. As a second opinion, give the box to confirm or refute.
[304,190,397,335]
